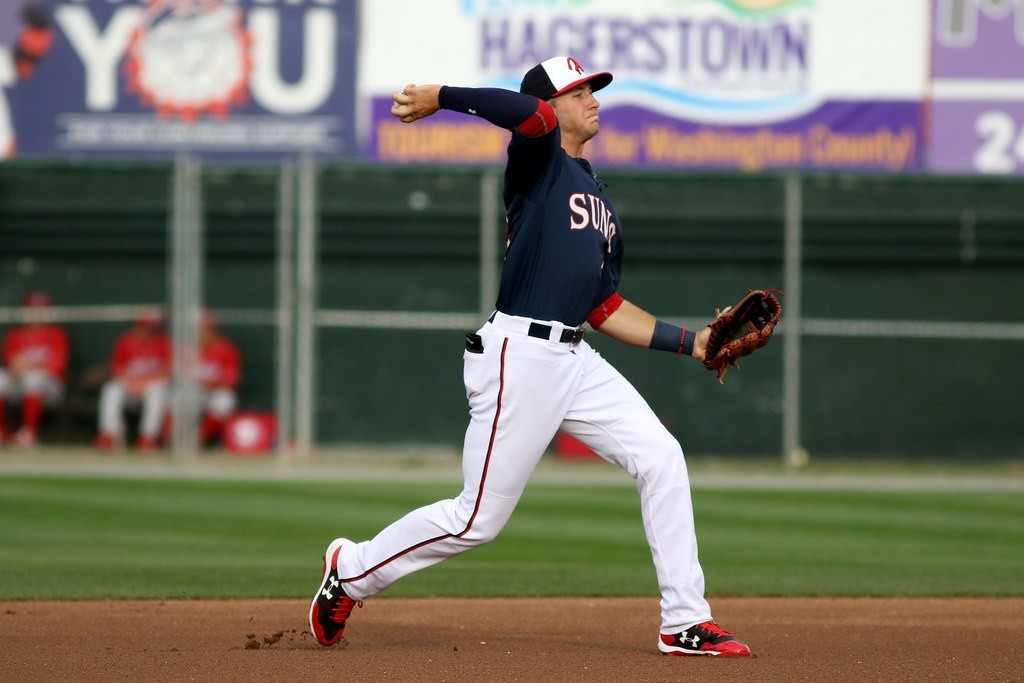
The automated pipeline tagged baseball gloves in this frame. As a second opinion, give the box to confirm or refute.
[704,286,783,370]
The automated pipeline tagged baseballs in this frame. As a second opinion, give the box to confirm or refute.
[392,91,406,108]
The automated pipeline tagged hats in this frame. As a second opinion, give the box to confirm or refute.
[520,56,613,101]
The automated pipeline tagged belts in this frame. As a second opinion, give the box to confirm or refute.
[488,311,585,343]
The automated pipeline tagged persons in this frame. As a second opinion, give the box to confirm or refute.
[0,294,70,445]
[308,55,780,658]
[163,314,241,445]
[96,307,172,452]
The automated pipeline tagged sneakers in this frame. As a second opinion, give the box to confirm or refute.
[309,538,363,646]
[658,621,750,657]
